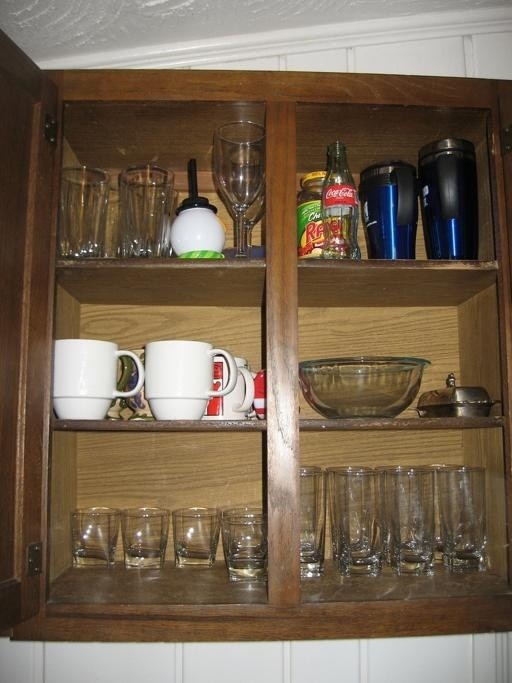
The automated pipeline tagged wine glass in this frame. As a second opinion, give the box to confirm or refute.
[208,119,267,260]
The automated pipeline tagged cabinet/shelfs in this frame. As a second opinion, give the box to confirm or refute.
[0,67,512,640]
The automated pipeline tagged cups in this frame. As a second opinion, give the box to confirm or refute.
[68,505,264,581]
[356,158,419,262]
[294,461,489,581]
[60,164,177,260]
[49,331,266,424]
[417,136,479,261]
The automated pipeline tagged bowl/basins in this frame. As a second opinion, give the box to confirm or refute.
[299,353,429,419]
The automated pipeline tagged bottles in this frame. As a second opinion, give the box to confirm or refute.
[324,140,362,262]
[169,157,227,259]
[295,169,352,259]
